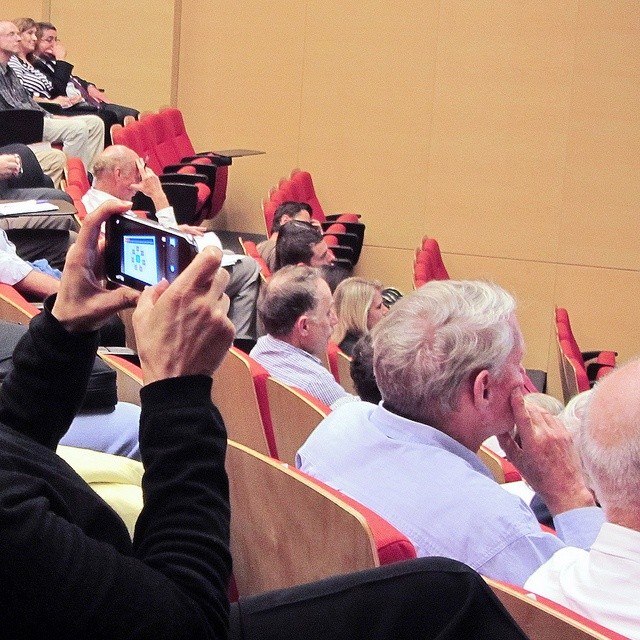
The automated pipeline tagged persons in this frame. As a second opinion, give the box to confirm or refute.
[0,215,76,302]
[0,19,142,216]
[249,265,352,414]
[523,358,640,635]
[257,200,313,270]
[530,388,603,529]
[274,220,334,278]
[294,277,614,589]
[295,331,379,473]
[1,198,527,636]
[331,277,388,356]
[81,144,259,338]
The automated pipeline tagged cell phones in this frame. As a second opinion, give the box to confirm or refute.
[104,212,200,292]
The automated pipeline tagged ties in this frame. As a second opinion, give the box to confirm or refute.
[39,53,102,109]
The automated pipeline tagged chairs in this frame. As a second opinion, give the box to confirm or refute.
[236,170,366,286]
[264,376,331,463]
[47,107,232,238]
[405,238,451,288]
[551,305,618,403]
[477,445,523,485]
[224,438,419,600]
[210,339,274,458]
[483,573,627,640]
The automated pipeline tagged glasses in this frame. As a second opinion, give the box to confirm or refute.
[42,36,60,42]
[0,34,22,40]
